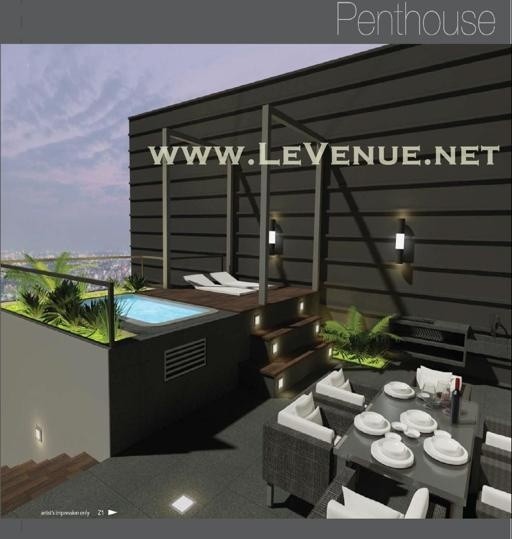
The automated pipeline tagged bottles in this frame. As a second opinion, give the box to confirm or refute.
[451,378,462,426]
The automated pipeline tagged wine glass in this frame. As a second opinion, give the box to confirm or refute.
[422,383,437,412]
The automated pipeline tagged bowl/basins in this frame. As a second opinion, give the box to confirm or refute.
[406,410,432,426]
[379,432,406,456]
[389,380,412,395]
[432,430,461,453]
[360,411,386,429]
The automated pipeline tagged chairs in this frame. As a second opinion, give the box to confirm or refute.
[175,269,279,297]
[263,368,511,518]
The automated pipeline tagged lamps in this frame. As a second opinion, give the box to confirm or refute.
[269,219,276,255]
[395,217,406,263]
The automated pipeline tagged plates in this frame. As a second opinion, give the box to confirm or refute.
[423,437,469,466]
[384,383,416,400]
[370,438,414,469]
[353,413,390,436]
[391,422,421,438]
[399,411,438,434]
